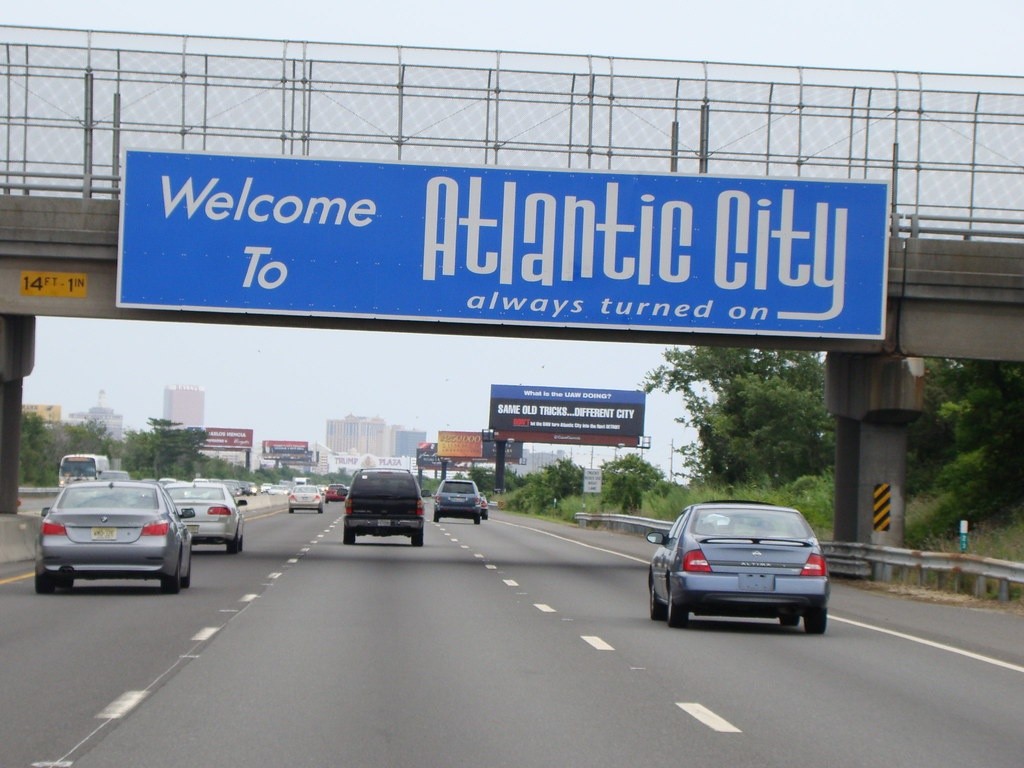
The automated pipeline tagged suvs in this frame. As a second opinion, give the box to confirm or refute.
[342,468,425,548]
[324,484,348,503]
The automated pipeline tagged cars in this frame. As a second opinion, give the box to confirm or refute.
[287,485,325,514]
[644,498,829,633]
[480,490,489,522]
[432,479,482,525]
[99,470,306,495]
[163,481,248,554]
[32,469,194,594]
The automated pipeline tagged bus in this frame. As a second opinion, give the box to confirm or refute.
[58,453,110,486]
